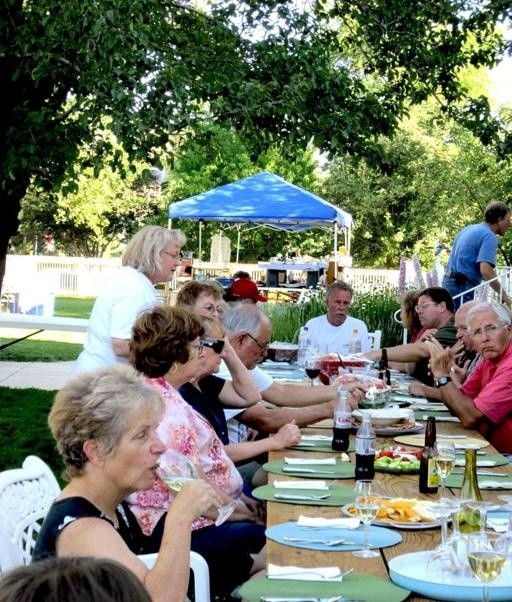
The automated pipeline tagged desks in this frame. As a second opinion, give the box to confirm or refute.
[256,261,326,289]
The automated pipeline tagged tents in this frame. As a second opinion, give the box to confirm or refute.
[165,169,353,294]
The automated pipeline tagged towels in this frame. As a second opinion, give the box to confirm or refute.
[299,433,333,441]
[454,460,494,467]
[284,454,337,467]
[453,440,479,449]
[394,395,428,406]
[273,479,328,492]
[422,416,461,422]
[266,562,344,583]
[297,513,361,531]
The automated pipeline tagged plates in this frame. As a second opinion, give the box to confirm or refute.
[342,498,454,530]
[388,550,512,601]
[354,422,423,434]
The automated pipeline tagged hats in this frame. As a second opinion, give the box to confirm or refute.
[230,279,267,302]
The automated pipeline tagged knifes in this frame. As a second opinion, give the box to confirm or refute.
[451,469,508,477]
[259,595,343,602]
[282,467,335,474]
[296,442,325,447]
[274,493,336,502]
[417,434,467,438]
[284,537,359,544]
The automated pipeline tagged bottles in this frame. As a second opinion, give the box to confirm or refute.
[453,447,486,531]
[330,390,352,450]
[354,411,380,481]
[298,327,311,363]
[419,416,441,493]
[378,350,391,391]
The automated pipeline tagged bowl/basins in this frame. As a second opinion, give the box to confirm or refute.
[317,355,369,385]
[335,375,390,408]
[352,408,409,426]
[268,347,298,362]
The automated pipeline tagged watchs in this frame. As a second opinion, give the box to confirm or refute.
[435,376,452,389]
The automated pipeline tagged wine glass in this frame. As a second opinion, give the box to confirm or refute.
[446,499,468,564]
[154,448,238,527]
[467,531,508,600]
[425,500,461,583]
[433,441,457,503]
[466,501,501,550]
[305,358,321,387]
[495,494,512,558]
[352,481,382,557]
[371,368,386,383]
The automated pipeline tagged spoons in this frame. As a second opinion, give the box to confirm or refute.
[292,539,345,546]
[306,494,332,499]
[265,567,354,580]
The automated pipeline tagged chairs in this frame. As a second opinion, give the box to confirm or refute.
[0,454,209,602]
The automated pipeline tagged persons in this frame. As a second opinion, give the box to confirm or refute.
[408,299,489,403]
[441,202,512,313]
[67,225,187,375]
[212,303,369,488]
[125,305,267,541]
[176,315,301,464]
[0,555,151,601]
[298,282,371,370]
[422,301,512,456]
[28,364,266,602]
[175,277,226,322]
[360,287,459,387]
[223,278,268,305]
[399,290,430,343]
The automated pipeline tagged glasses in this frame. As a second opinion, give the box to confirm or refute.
[201,339,225,354]
[413,302,439,312]
[186,343,205,354]
[162,250,184,260]
[469,324,508,336]
[245,332,272,353]
